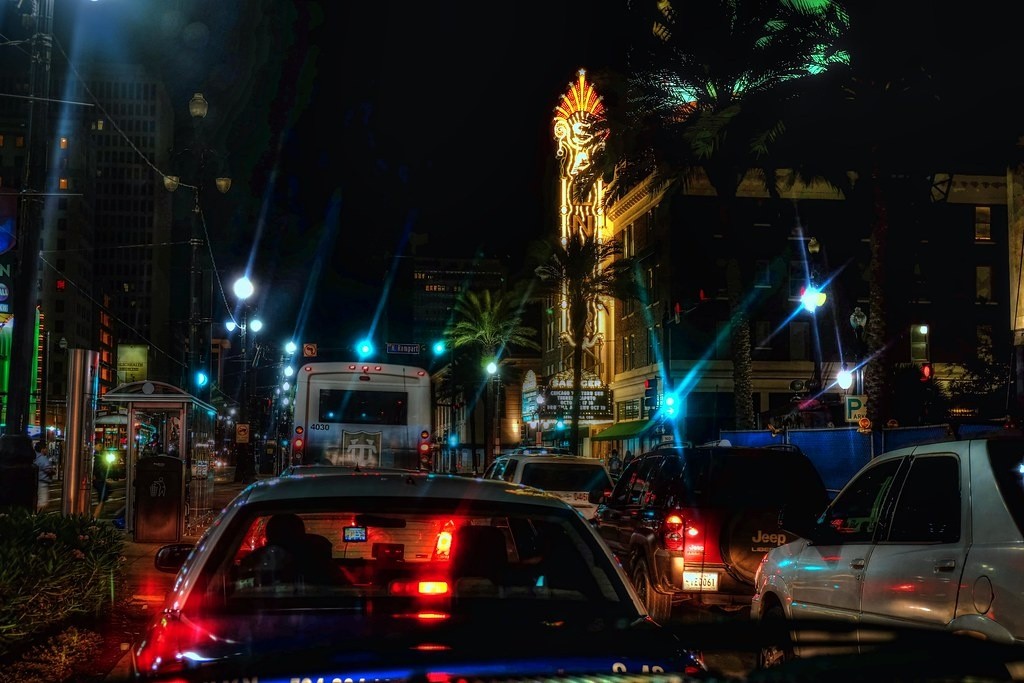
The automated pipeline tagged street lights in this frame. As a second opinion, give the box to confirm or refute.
[163,94,236,539]
[848,307,867,394]
[226,268,265,472]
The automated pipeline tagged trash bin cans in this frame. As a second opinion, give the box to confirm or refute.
[133,453,191,544]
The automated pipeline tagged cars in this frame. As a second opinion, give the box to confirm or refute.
[192,460,208,479]
[751,434,1023,683]
[125,473,709,683]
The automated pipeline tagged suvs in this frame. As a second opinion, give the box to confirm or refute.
[588,441,833,631]
[481,453,614,521]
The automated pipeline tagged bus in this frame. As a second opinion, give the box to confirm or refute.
[288,362,433,474]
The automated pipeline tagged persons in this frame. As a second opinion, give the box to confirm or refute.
[607,449,634,475]
[34,441,55,512]
[92,438,111,518]
[231,514,352,589]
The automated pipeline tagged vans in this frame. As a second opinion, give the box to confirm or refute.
[496,447,575,456]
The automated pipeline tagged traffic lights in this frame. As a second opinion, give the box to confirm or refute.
[262,397,270,413]
[664,392,675,414]
[922,364,932,382]
[43,271,75,310]
[644,378,657,407]
[699,286,717,301]
[675,299,689,317]
